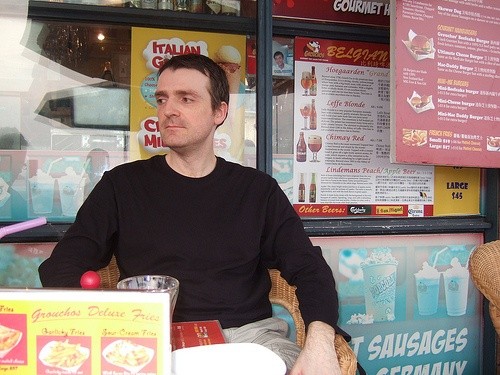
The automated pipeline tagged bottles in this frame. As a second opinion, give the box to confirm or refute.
[309,173,316,202]
[309,99,317,130]
[298,173,305,202]
[296,132,306,162]
[310,65,317,96]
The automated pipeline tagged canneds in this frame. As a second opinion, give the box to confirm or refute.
[130,0,203,13]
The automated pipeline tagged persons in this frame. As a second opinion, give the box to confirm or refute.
[272,50,293,76]
[37,54,343,375]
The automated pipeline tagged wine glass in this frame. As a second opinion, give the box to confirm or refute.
[300,109,310,130]
[301,72,312,96]
[308,136,322,162]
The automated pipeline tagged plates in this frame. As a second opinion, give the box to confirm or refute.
[172,343,287,375]
[0,325,22,358]
[39,341,89,372]
[103,341,154,373]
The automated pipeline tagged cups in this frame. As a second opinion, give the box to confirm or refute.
[116,275,179,327]
[415,273,441,315]
[58,178,86,216]
[443,273,469,316]
[29,178,55,213]
[362,263,396,321]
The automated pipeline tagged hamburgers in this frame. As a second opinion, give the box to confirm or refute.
[411,34,431,55]
[411,95,432,108]
[106,340,149,366]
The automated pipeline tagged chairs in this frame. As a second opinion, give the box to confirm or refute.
[469,239,500,335]
[96,252,357,375]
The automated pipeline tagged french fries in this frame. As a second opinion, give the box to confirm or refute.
[45,338,87,368]
